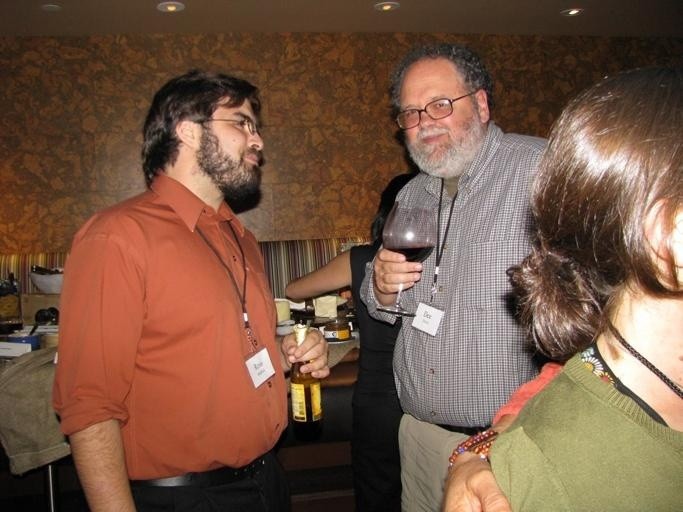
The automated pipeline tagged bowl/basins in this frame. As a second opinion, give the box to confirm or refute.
[29,267,65,295]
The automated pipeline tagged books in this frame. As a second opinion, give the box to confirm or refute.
[0,336,41,359]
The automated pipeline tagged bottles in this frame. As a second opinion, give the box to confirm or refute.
[289,325,324,446]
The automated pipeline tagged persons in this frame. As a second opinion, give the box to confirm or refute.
[485,63,682,512]
[443,351,560,512]
[356,41,556,512]
[283,174,418,511]
[50,67,333,512]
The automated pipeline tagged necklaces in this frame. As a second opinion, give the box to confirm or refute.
[605,315,683,401]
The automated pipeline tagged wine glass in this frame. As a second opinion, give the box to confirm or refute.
[376,199,437,316]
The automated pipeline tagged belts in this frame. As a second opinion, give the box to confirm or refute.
[437,424,490,436]
[128,446,275,490]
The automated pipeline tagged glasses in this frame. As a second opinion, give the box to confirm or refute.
[394,89,477,130]
[199,118,261,136]
